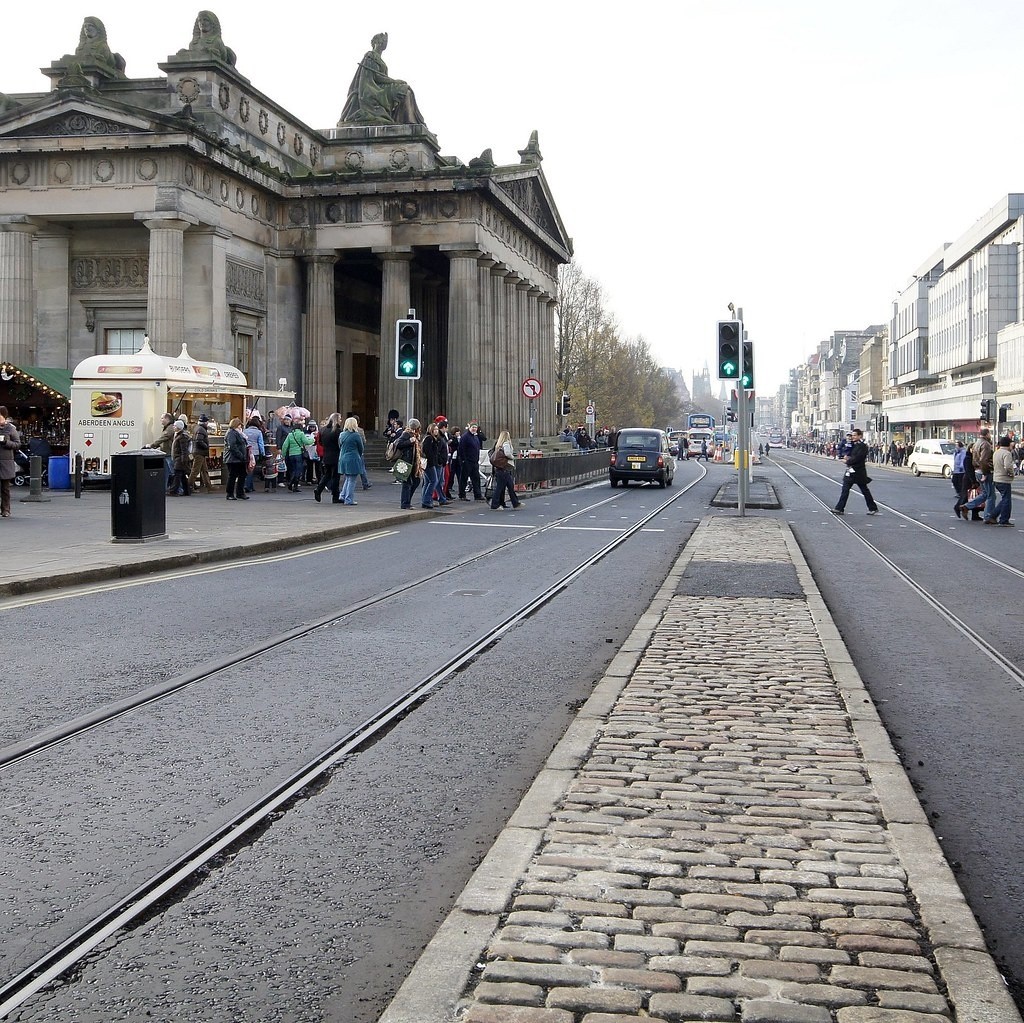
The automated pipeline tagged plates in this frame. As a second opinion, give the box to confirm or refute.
[91,398,121,416]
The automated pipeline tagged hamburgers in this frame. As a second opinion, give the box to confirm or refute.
[93,394,119,414]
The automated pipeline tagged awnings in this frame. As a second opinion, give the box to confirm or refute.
[169,384,294,398]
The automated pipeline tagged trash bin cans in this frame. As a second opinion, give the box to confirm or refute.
[109,449,167,539]
[48,456,72,489]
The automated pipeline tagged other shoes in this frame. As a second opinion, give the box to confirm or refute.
[167,485,220,497]
[867,509,879,515]
[954,504,1016,527]
[226,475,373,506]
[2,510,10,516]
[401,495,528,511]
[831,508,845,516]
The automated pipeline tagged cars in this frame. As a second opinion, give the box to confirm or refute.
[906,439,957,479]
[609,429,675,488]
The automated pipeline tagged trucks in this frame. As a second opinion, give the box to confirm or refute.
[69,336,299,479]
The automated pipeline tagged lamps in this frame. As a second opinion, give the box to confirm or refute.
[211,369,219,385]
[278,377,288,392]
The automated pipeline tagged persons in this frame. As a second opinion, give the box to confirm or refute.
[787,440,843,466]
[951,429,1024,526]
[223,407,372,505]
[383,410,526,510]
[837,431,855,475]
[0,405,21,517]
[677,433,684,460]
[864,441,914,467]
[145,412,215,496]
[831,428,878,514]
[765,442,770,455]
[564,425,617,451]
[697,437,709,461]
[759,442,763,455]
[683,434,690,460]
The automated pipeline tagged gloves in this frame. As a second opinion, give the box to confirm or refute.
[0,437,7,446]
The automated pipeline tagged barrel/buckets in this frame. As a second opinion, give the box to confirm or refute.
[48,455,72,489]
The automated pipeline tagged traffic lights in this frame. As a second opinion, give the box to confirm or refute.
[561,395,572,417]
[980,400,990,422]
[736,341,754,391]
[727,407,733,421]
[717,320,743,380]
[395,320,422,380]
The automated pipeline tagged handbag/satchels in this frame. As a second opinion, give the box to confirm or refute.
[392,458,413,481]
[300,447,308,457]
[485,475,495,498]
[967,484,986,508]
[276,454,288,472]
[488,442,510,469]
[306,444,319,460]
[386,431,412,461]
[246,445,256,472]
[419,457,428,470]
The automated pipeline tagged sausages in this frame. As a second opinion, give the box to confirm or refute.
[206,456,223,470]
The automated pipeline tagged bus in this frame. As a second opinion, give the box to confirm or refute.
[667,431,688,454]
[715,425,730,450]
[688,428,716,459]
[768,427,783,448]
[688,414,715,430]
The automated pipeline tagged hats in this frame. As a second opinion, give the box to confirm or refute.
[1001,437,1015,447]
[308,419,317,426]
[175,420,184,430]
[284,414,291,420]
[409,418,421,429]
[177,413,187,422]
[469,420,478,428]
[435,416,446,424]
[198,413,209,422]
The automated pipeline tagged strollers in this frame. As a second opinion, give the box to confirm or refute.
[13,448,49,486]
[465,469,488,492]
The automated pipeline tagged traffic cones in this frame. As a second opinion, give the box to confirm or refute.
[714,447,722,461]
